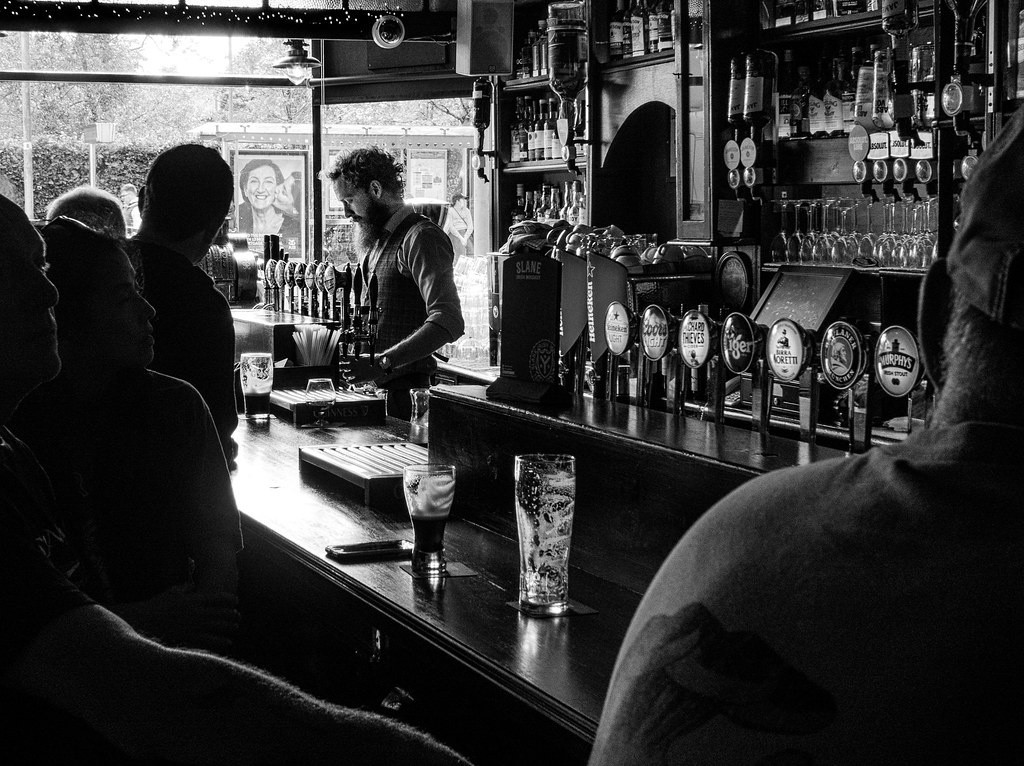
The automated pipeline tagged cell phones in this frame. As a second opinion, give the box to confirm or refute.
[325,540,413,560]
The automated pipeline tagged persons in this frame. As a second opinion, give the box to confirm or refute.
[324,145,465,420]
[0,193,476,766]
[5,143,245,667]
[238,158,302,258]
[584,104,1024,765]
[273,171,302,213]
[446,192,474,268]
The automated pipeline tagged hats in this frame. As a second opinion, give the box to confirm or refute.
[452,193,468,202]
[948,105,1024,331]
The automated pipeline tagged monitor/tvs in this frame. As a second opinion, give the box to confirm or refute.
[748,264,858,333]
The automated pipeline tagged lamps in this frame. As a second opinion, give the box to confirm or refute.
[273,37,322,85]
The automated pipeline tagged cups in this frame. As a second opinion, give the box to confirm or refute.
[356,385,388,415]
[408,387,429,425]
[514,452,577,616]
[239,353,274,418]
[499,220,708,268]
[342,354,377,392]
[401,463,457,574]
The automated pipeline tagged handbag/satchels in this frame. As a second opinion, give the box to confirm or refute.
[470,230,475,238]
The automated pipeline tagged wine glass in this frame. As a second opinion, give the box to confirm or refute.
[768,196,938,270]
[304,377,337,426]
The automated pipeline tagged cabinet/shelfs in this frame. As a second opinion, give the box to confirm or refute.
[489,0,1024,450]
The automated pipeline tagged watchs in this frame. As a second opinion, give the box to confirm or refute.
[376,353,394,376]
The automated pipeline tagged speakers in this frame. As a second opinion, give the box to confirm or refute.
[456,0,514,77]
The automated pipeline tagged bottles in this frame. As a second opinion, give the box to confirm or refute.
[944,0,987,73]
[721,54,769,144]
[509,179,586,228]
[547,3,589,141]
[508,94,585,160]
[521,19,548,79]
[880,0,923,73]
[608,0,675,59]
[773,41,951,139]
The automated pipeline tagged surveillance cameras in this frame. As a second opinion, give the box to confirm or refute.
[372,15,405,49]
